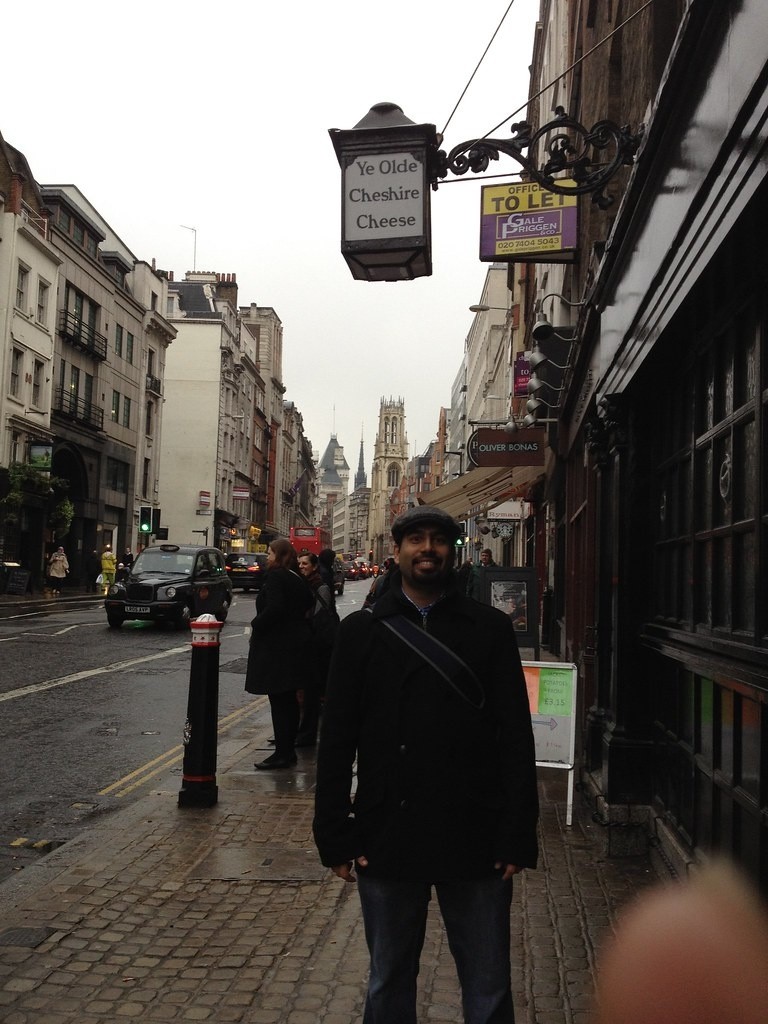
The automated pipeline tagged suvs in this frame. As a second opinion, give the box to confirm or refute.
[103,544,234,631]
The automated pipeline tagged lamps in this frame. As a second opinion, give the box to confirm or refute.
[469,305,510,312]
[220,413,243,419]
[526,378,564,413]
[531,292,587,340]
[492,525,499,538]
[328,101,643,282]
[530,331,580,369]
[503,415,517,432]
[523,397,561,428]
[527,359,572,398]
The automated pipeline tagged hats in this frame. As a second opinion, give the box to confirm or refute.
[391,505,462,543]
[318,548,335,565]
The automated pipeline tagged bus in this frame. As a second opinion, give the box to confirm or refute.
[289,527,332,559]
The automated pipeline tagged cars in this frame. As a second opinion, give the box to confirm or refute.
[225,552,269,593]
[332,551,371,595]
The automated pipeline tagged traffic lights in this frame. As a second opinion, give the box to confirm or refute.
[139,506,153,534]
[455,522,467,548]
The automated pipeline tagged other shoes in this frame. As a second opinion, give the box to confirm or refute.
[255,752,297,769]
[295,740,317,747]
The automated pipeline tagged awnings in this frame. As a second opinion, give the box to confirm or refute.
[413,431,555,525]
[219,506,248,528]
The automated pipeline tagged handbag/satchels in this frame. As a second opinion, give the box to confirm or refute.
[304,607,337,651]
[96,574,104,583]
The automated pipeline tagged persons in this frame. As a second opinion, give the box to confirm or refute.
[97,543,117,590]
[115,563,128,582]
[465,548,501,604]
[48,546,69,594]
[120,548,134,568]
[317,547,337,615]
[309,503,541,1023]
[362,558,482,612]
[242,537,313,771]
[299,552,340,703]
